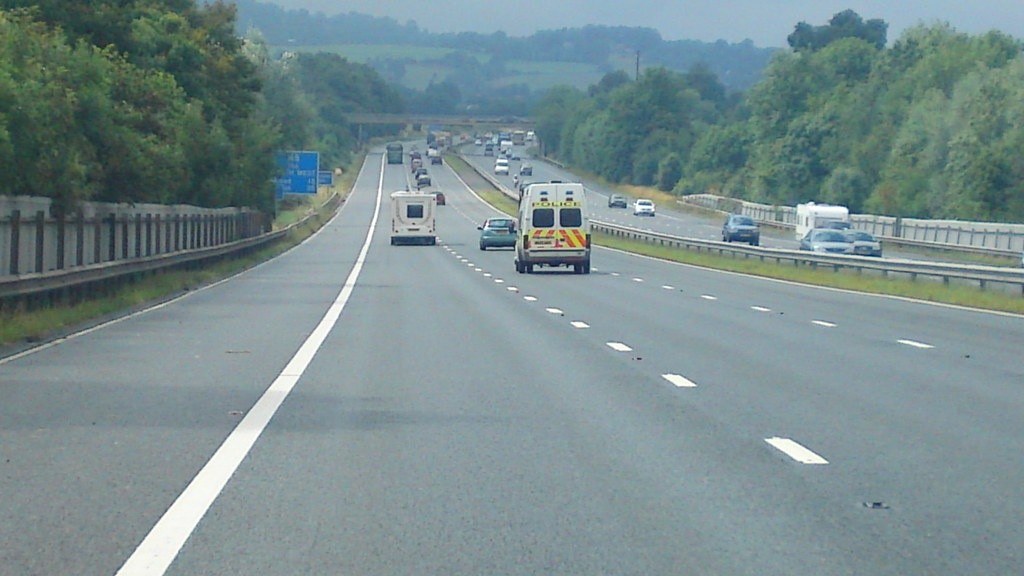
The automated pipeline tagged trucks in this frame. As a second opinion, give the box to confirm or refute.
[795,202,849,239]
[391,191,436,245]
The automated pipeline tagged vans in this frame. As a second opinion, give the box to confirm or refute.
[514,180,591,276]
[495,159,509,175]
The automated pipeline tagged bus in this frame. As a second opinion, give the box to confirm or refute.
[386,141,403,164]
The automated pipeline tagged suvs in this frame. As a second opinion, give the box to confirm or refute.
[723,216,759,246]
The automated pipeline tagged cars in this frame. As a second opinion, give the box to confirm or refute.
[459,125,536,161]
[608,194,626,208]
[410,131,452,204]
[840,228,882,257]
[477,218,516,250]
[633,199,655,217]
[520,165,532,176]
[799,226,854,255]
